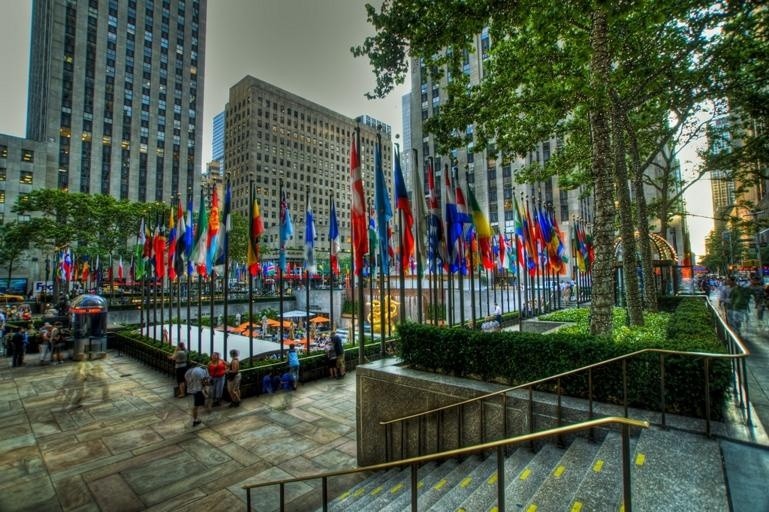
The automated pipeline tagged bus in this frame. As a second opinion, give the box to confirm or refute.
[0,277,28,302]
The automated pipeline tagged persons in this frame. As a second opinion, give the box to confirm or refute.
[695,271,769,340]
[268,371,288,408]
[166,343,188,398]
[324,340,338,378]
[206,352,230,408]
[260,370,273,405]
[226,349,241,408]
[1,304,72,367]
[364,276,581,363]
[287,344,301,391]
[281,367,297,405]
[183,363,208,426]
[329,331,346,380]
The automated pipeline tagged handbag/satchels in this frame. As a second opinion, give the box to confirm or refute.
[174,385,186,398]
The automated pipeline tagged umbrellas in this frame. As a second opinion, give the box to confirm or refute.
[216,308,330,345]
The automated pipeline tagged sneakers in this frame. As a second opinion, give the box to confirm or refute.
[211,400,241,409]
[193,420,201,427]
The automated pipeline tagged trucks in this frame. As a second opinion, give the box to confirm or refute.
[32,280,85,299]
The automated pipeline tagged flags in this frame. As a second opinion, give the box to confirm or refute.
[55,130,595,284]
